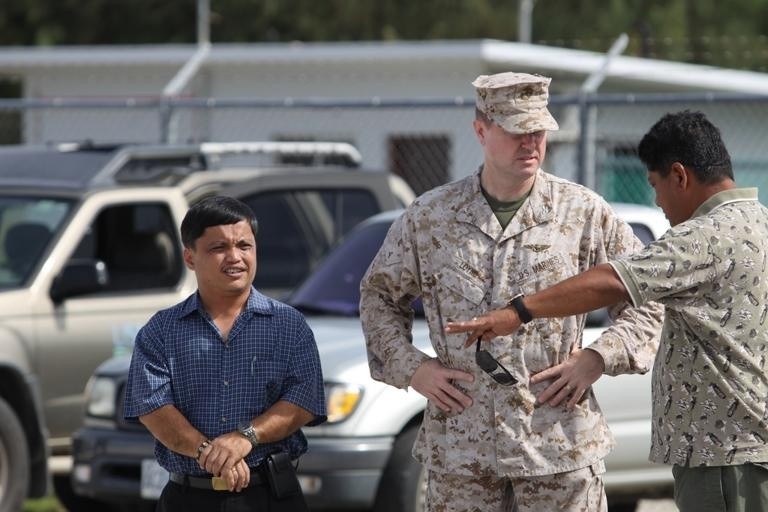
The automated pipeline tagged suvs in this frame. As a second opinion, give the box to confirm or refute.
[0,139,417,512]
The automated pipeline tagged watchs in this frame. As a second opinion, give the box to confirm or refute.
[238,421,258,448]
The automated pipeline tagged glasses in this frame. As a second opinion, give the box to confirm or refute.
[474,337,518,386]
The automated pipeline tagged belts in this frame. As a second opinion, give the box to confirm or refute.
[167,471,262,491]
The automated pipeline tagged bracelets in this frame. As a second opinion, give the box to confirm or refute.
[508,294,532,323]
[195,439,211,463]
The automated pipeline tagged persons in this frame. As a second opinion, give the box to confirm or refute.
[358,70,666,512]
[444,109,767,512]
[123,196,328,511]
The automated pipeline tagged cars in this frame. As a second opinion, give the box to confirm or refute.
[71,202,677,512]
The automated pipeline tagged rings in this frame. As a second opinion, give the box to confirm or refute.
[567,385,574,391]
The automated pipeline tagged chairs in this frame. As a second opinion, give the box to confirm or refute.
[100,229,179,290]
[5,222,53,265]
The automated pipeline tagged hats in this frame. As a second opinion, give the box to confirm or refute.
[473,71,560,135]
